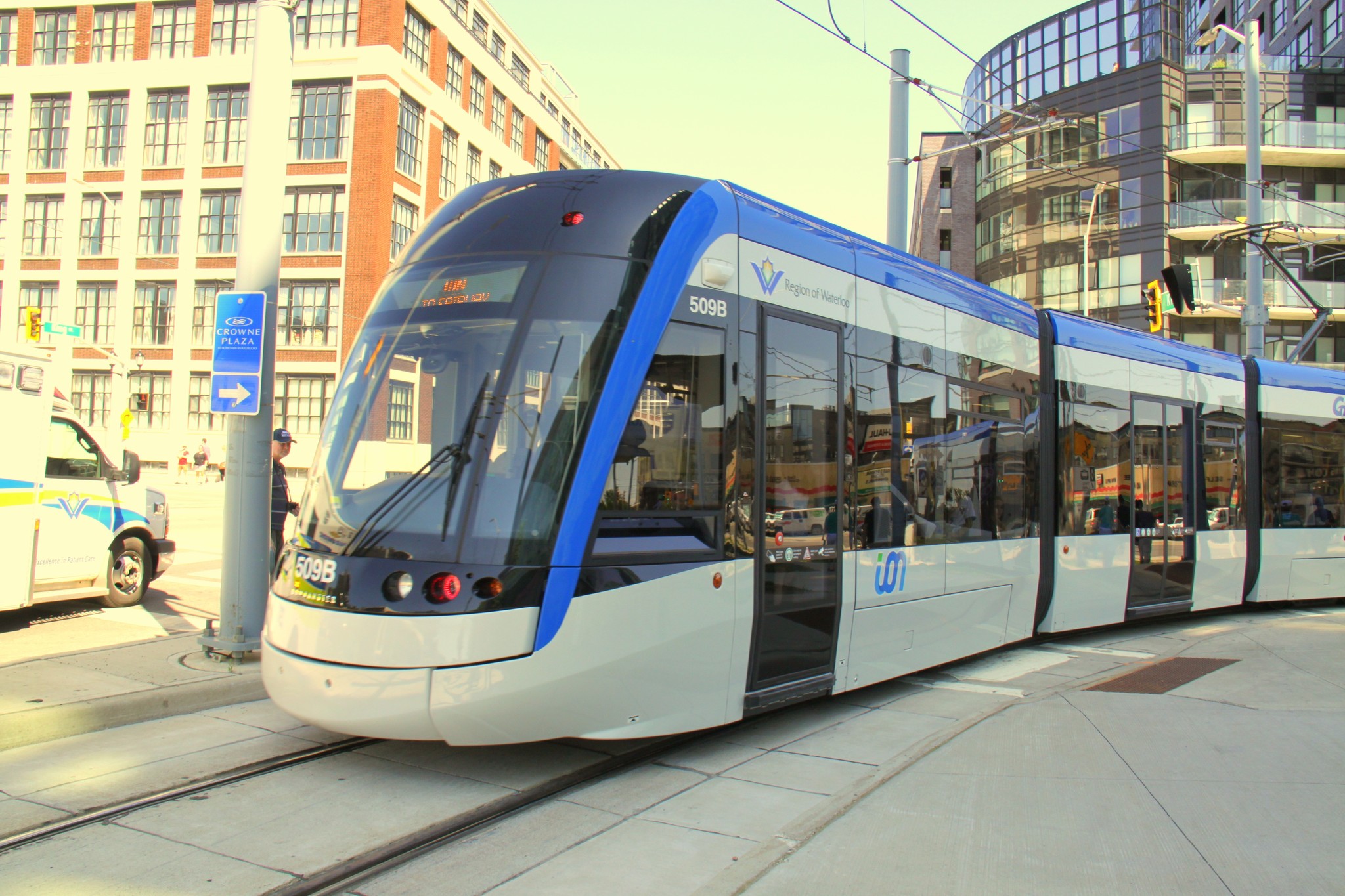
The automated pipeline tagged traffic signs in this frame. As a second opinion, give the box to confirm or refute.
[209,291,267,417]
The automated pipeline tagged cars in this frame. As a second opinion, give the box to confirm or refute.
[1084,507,1099,536]
[1158,506,1238,541]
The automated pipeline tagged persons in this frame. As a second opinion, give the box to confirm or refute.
[947,482,976,538]
[269,427,299,582]
[864,495,892,549]
[983,496,1007,532]
[823,499,836,570]
[1273,500,1303,528]
[1098,497,1115,534]
[1136,498,1156,564]
[1118,494,1131,534]
[1306,495,1337,528]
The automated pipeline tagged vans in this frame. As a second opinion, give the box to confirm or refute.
[764,503,907,538]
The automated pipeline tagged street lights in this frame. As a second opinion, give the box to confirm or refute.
[134,349,145,426]
[1191,19,1266,358]
[70,175,126,450]
[1083,182,1107,318]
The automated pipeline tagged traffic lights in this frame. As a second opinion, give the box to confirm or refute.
[26,307,41,341]
[1140,279,1163,332]
[136,393,149,411]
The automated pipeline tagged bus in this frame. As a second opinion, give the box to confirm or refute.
[262,167,1345,748]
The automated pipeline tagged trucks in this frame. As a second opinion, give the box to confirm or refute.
[0,338,177,613]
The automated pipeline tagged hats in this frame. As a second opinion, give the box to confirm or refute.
[273,428,297,444]
